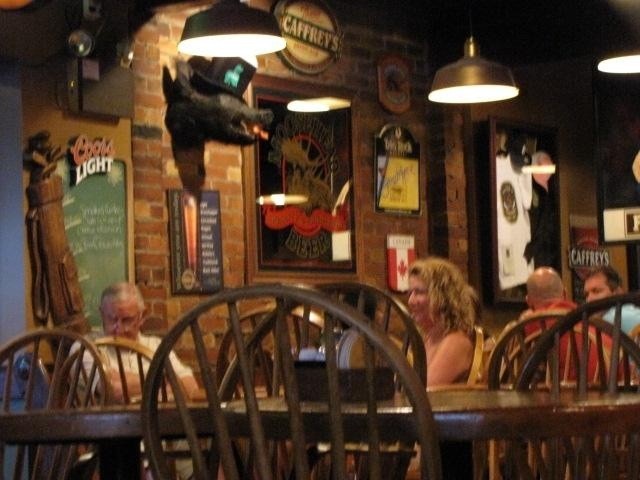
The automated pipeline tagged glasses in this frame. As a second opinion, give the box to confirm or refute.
[101,320,138,334]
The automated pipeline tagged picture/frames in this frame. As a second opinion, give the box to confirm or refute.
[372,122,423,218]
[242,73,365,288]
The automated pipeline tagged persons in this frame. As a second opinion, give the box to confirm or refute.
[583,264,640,359]
[68,283,197,479]
[511,267,627,385]
[398,257,476,480]
[301,291,378,369]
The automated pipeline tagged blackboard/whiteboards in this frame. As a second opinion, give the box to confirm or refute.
[23,117,135,365]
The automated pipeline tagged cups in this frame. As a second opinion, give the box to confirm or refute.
[297,345,326,363]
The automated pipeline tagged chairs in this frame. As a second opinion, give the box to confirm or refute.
[0,279,639,480]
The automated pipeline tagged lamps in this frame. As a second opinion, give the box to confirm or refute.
[427,9,519,104]
[177,0,287,57]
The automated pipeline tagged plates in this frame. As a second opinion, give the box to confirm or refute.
[336,327,379,369]
